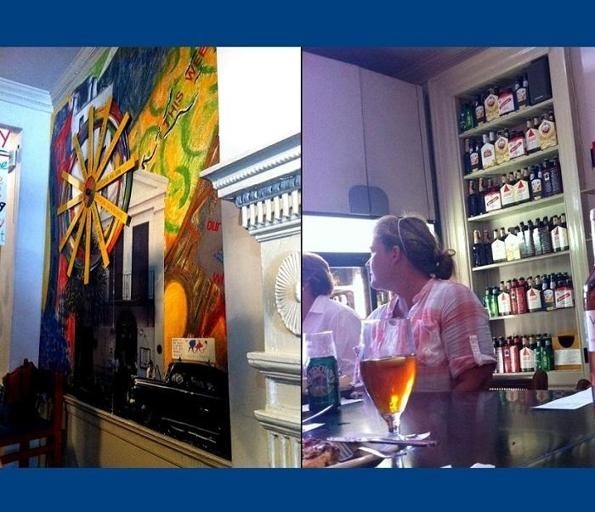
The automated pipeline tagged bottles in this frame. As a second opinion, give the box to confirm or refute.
[581,207,595,407]
[456,78,573,375]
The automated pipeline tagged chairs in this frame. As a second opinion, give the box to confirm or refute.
[0,360,65,468]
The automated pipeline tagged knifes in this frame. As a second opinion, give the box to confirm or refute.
[327,435,437,449]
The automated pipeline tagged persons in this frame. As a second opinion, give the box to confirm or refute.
[351,215,498,392]
[400,394,499,467]
[300,252,362,376]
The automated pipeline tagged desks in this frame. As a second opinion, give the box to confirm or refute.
[302,389,595,468]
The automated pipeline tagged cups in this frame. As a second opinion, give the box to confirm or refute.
[303,329,344,378]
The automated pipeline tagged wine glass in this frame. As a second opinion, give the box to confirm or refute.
[357,316,424,459]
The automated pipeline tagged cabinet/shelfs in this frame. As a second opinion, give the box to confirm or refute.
[450,51,588,386]
